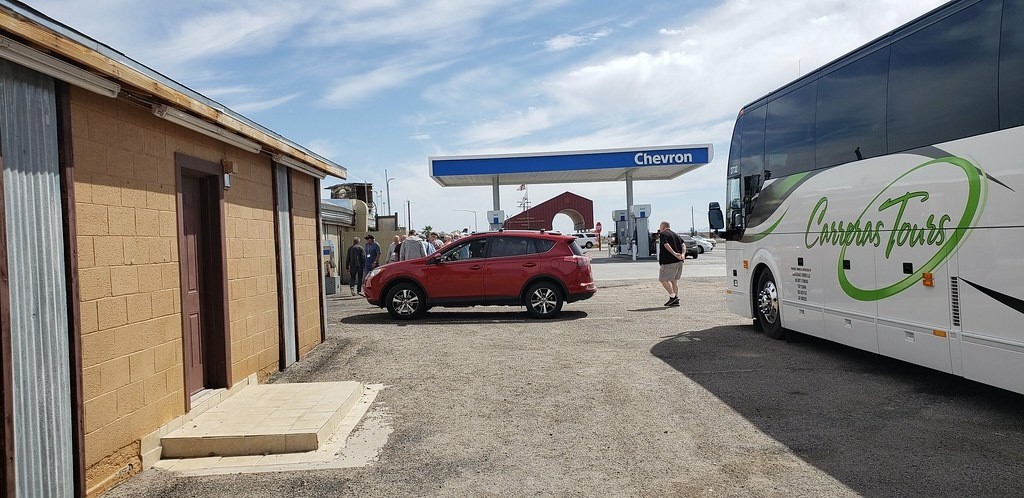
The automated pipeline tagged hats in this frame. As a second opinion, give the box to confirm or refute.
[363,234,376,240]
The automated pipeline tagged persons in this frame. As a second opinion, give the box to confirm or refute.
[359,234,382,298]
[659,220,687,307]
[419,227,544,264]
[656,229,660,239]
[394,234,407,262]
[345,236,365,296]
[385,234,401,264]
[399,229,427,261]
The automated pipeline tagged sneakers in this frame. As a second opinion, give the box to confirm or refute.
[664,296,681,306]
[350,287,355,296]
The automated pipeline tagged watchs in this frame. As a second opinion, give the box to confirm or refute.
[373,261,379,264]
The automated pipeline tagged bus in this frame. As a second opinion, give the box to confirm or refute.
[706,0,1024,395]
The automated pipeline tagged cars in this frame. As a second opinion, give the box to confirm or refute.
[569,233,600,250]
[678,234,698,260]
[364,228,597,319]
[692,237,713,254]
[698,236,716,247]
